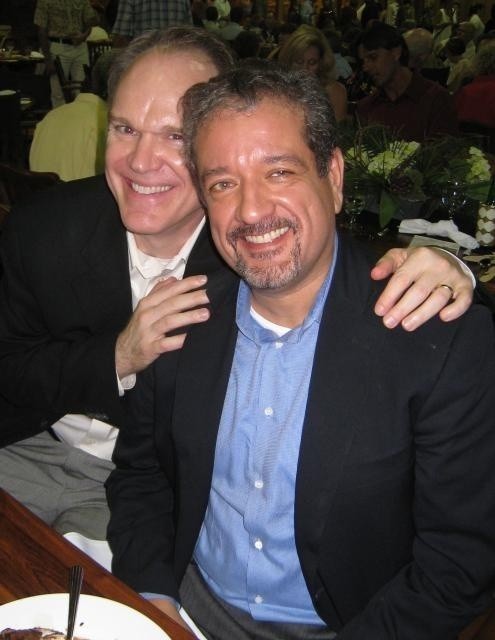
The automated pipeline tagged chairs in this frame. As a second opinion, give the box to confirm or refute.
[1,30,134,211]
[333,46,475,118]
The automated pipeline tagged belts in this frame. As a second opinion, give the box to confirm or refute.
[50,38,70,44]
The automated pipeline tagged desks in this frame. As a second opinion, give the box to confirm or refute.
[0,483,199,640]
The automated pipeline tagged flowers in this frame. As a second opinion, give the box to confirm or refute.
[338,112,494,228]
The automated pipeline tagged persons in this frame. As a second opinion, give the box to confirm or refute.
[0,0,495,144]
[104,66,495,640]
[29,50,116,182]
[0,28,478,541]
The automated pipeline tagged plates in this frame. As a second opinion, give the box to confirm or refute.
[0,593,171,639]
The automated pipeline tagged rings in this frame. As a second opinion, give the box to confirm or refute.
[435,284,454,300]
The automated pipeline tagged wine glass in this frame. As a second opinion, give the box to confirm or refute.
[442,181,467,219]
[343,179,365,224]
